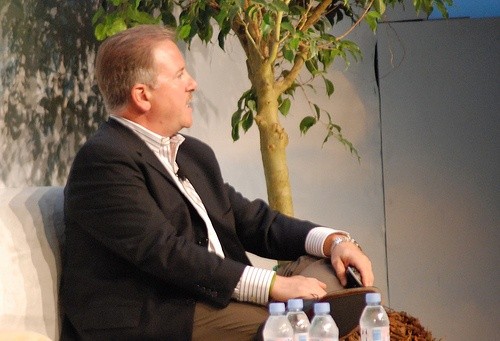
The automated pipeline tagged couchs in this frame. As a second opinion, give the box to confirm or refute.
[0,178,64,341]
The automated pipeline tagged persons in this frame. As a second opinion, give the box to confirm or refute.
[60,26,374,341]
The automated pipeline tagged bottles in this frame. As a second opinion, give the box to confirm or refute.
[262,302,293,341]
[308,303,339,341]
[287,299,310,341]
[359,293,390,341]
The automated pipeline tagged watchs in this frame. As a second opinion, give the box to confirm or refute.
[330,236,364,257]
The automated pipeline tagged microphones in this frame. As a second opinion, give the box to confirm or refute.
[177,167,186,181]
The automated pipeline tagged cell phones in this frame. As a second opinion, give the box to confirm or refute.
[348,266,363,286]
